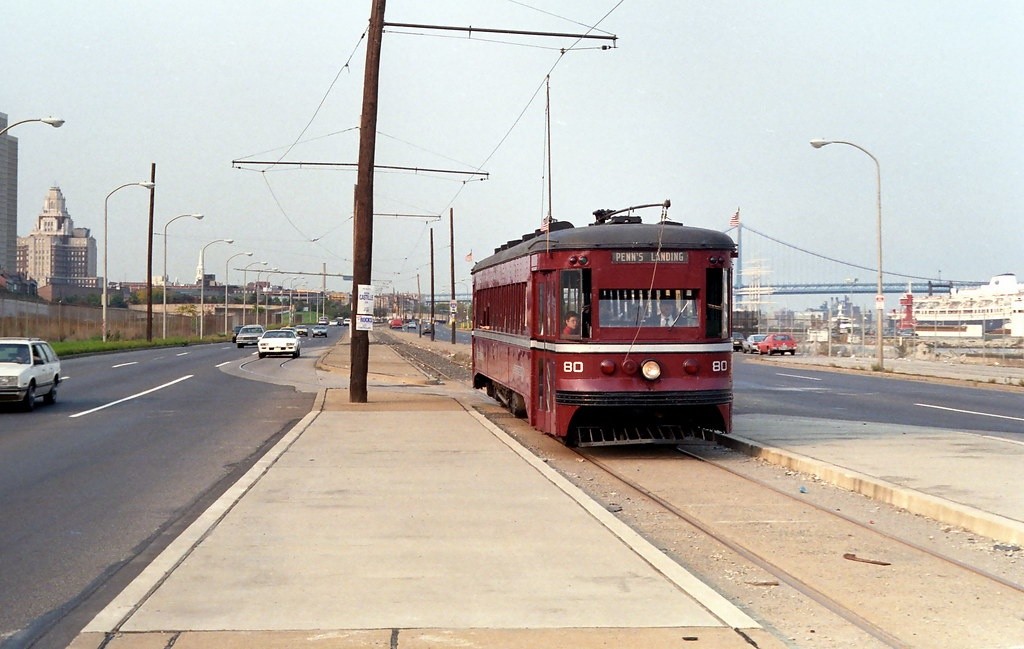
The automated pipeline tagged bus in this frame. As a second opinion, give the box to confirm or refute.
[468,201,740,451]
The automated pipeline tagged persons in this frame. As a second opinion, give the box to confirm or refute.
[563,310,580,334]
[9,346,42,364]
[646,295,687,327]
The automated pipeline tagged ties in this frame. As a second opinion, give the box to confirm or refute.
[665,318,670,326]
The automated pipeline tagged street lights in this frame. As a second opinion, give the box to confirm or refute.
[809,138,885,371]
[242,260,269,326]
[256,266,333,325]
[101,181,156,343]
[161,214,205,339]
[223,251,254,336]
[198,238,235,340]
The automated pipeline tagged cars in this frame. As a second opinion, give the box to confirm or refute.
[731,332,747,352]
[407,322,417,328]
[741,334,767,354]
[757,333,798,357]
[0,336,62,412]
[235,323,266,349]
[311,325,328,338]
[421,322,432,334]
[387,317,404,329]
[317,317,351,327]
[231,325,244,343]
[256,324,307,358]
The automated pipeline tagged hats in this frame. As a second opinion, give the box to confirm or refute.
[659,296,676,305]
